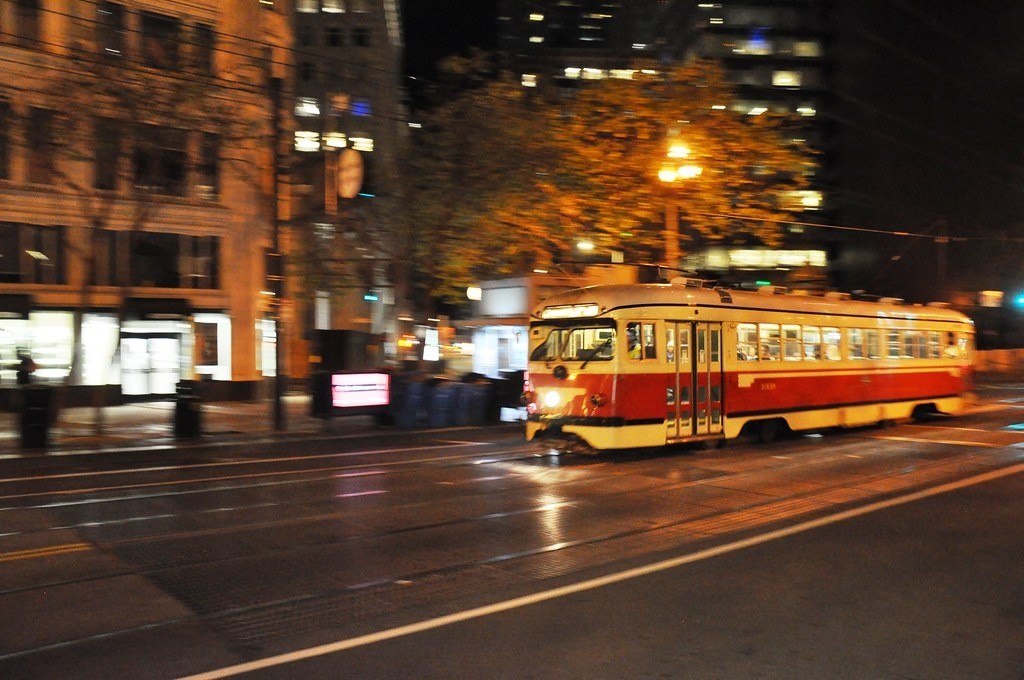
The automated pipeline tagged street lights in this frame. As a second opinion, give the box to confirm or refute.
[659,133,702,283]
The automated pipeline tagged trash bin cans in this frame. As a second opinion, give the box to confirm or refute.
[174,380,202,438]
[390,373,509,429]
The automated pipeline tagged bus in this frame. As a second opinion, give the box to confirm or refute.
[520,277,978,452]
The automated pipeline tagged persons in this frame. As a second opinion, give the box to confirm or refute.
[756,345,771,359]
[15,359,37,385]
[666,340,674,362]
[627,329,641,359]
[813,345,829,360]
